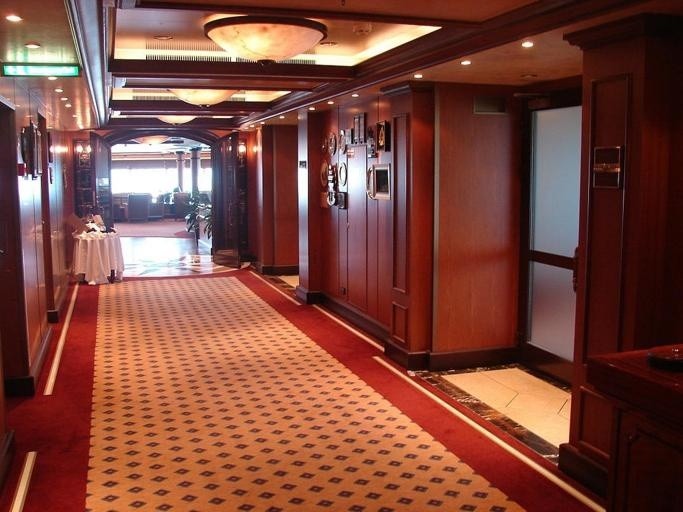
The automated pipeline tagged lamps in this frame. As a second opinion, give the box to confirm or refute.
[166,89,241,110]
[157,116,196,126]
[203,13,328,68]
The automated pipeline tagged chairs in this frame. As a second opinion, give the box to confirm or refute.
[113,192,191,223]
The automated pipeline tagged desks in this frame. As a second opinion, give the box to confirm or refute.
[72,232,125,285]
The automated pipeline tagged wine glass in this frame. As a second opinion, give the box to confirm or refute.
[87,214,94,229]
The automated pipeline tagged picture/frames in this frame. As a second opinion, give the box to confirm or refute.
[316,112,392,209]
